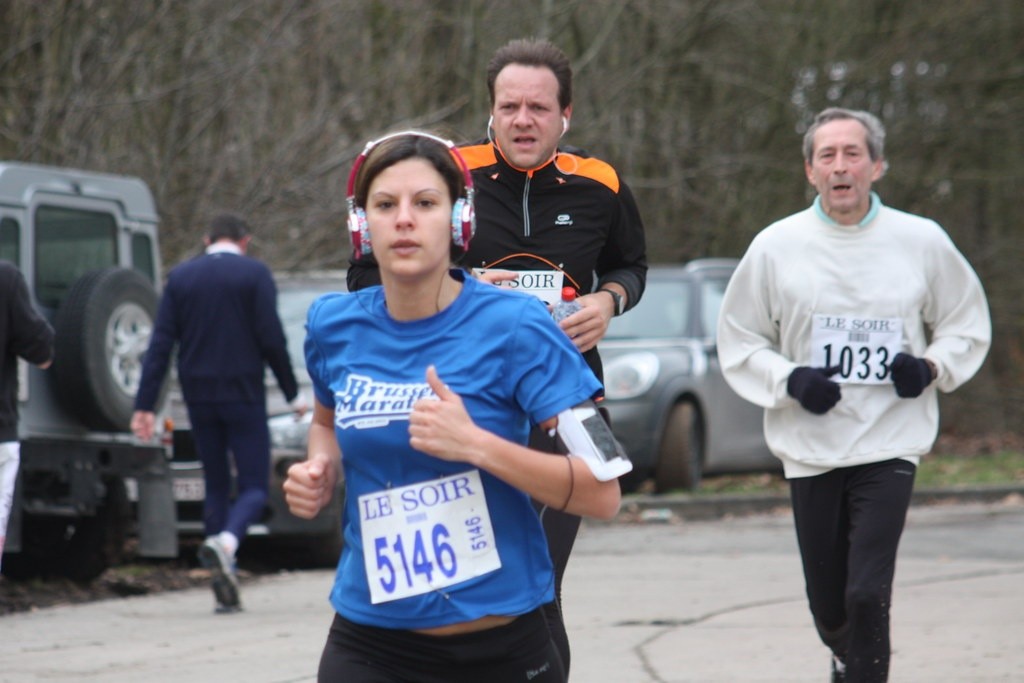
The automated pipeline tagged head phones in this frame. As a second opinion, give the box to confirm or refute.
[347,131,477,257]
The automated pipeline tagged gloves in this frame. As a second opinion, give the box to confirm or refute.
[889,352,931,400]
[785,365,844,415]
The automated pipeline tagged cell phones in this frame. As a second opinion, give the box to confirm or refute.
[574,406,622,466]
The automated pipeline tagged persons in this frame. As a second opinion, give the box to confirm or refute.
[347,36,649,614]
[0,262,55,557]
[283,130,621,683]
[717,107,993,683]
[130,215,298,613]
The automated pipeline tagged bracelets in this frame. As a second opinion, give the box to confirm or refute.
[558,454,574,513]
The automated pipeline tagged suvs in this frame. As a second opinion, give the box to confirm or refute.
[592,256,786,500]
[1,161,180,590]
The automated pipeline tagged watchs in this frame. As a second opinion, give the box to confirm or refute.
[596,288,624,316]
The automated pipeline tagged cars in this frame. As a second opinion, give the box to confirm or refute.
[162,267,346,569]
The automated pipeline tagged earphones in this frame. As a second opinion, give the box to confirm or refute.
[562,116,568,130]
[488,115,494,127]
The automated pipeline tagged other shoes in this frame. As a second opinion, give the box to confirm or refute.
[198,537,239,608]
